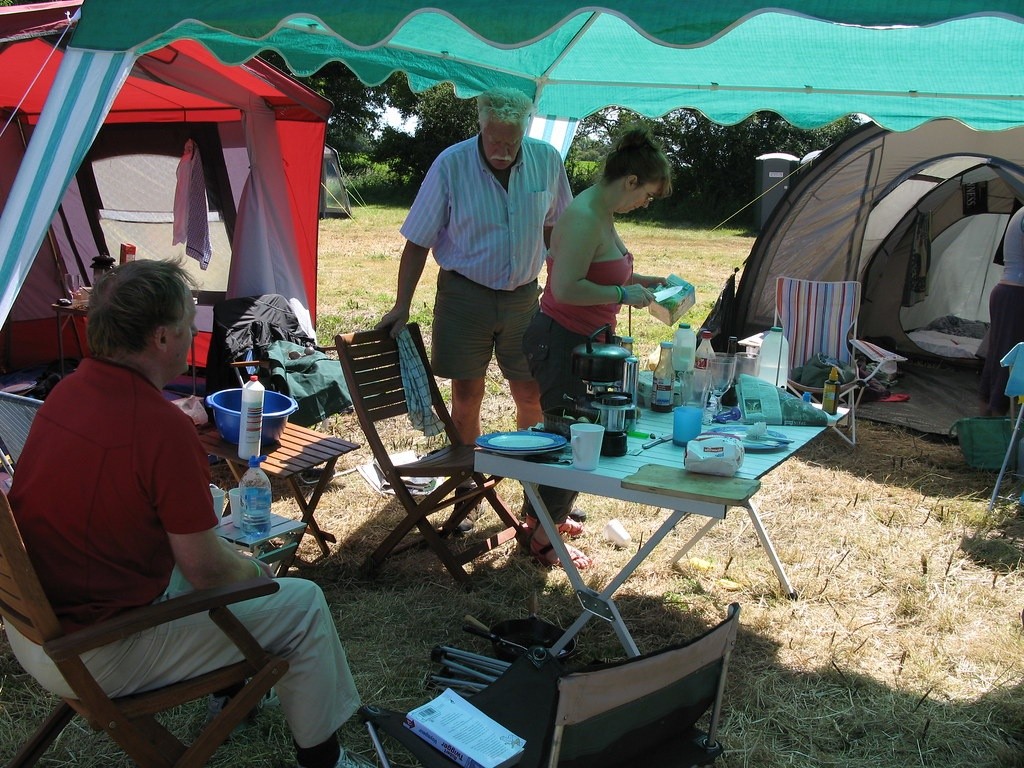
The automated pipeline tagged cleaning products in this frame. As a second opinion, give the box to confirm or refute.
[238,375,265,460]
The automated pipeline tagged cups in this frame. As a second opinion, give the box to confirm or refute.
[679,369,711,411]
[78,287,92,300]
[735,352,760,417]
[210,488,225,525]
[605,518,632,546]
[673,407,703,447]
[229,488,241,528]
[570,424,605,470]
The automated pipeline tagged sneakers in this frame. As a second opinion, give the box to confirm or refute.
[336,746,378,768]
[208,694,234,713]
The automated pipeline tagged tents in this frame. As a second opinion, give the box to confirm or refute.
[0,0,367,394]
[0,0,1024,333]
[734,122,1024,437]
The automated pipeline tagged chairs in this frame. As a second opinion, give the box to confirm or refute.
[334,321,530,593]
[751,277,908,448]
[354,601,741,767]
[988,342,1024,511]
[0,434,297,768]
[214,294,353,416]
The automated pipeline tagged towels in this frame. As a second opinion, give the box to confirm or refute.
[172,137,194,247]
[395,323,446,437]
[185,144,213,272]
[999,341,1024,404]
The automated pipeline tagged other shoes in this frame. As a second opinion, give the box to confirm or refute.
[443,491,485,534]
[570,509,587,521]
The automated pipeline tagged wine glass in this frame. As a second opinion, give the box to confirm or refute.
[706,353,736,422]
[65,275,80,307]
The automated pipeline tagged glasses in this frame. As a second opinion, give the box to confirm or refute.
[640,182,653,203]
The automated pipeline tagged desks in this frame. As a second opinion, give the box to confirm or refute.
[197,421,360,572]
[475,386,852,660]
[54,300,197,397]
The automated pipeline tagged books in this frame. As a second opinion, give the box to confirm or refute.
[403,687,527,768]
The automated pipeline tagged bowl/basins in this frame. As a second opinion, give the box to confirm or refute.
[206,388,299,444]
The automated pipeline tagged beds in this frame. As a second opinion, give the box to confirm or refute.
[900,313,993,367]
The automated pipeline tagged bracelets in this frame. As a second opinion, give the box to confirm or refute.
[615,285,628,303]
[248,559,262,578]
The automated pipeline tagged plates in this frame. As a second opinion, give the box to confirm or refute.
[476,431,567,455]
[707,426,788,448]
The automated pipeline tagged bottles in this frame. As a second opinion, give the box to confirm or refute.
[823,368,840,415]
[651,342,675,412]
[721,337,738,406]
[759,327,789,392]
[803,392,811,403]
[238,376,265,460]
[621,337,632,354]
[239,455,272,537]
[673,323,696,382]
[695,331,715,369]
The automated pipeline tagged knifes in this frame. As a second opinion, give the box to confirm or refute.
[642,434,673,448]
[653,286,683,302]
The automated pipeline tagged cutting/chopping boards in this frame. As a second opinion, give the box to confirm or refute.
[621,464,761,506]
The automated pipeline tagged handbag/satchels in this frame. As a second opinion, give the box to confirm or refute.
[948,416,1016,470]
[260,340,354,428]
[800,352,854,388]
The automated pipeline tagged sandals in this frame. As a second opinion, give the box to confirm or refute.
[529,536,592,569]
[522,513,583,536]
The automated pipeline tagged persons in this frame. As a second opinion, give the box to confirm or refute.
[978,206,1024,417]
[520,124,673,569]
[375,85,587,531]
[16,281,381,768]
